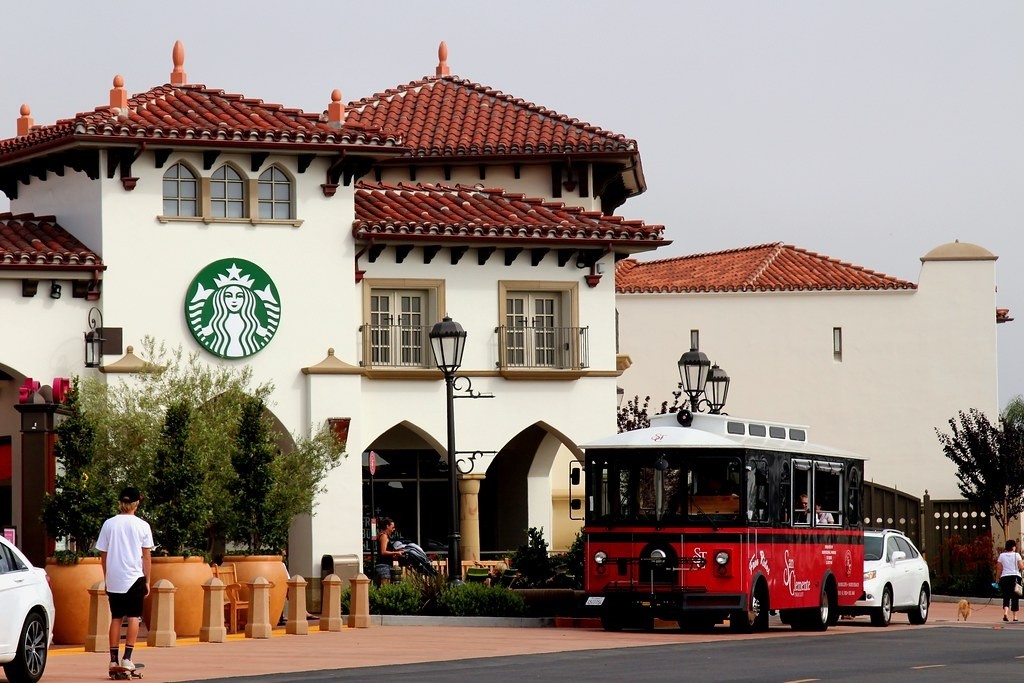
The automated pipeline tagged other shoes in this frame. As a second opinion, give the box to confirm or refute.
[1003,614,1009,621]
[1013,618,1018,621]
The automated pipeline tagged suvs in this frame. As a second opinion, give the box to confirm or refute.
[829,530,931,627]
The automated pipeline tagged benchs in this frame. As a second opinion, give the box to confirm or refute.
[209,561,249,634]
[402,558,449,582]
[461,558,510,582]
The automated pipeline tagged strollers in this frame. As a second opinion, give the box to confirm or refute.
[400,544,443,585]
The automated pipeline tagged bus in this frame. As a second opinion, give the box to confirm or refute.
[569,409,865,634]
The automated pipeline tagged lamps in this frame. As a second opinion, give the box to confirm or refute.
[82,306,107,369]
[597,263,605,273]
[575,255,586,268]
[51,283,62,298]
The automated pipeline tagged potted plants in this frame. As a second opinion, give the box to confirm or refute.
[98,334,271,638]
[38,368,151,645]
[219,373,350,631]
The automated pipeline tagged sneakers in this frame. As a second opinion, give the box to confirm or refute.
[109,662,119,670]
[121,659,135,670]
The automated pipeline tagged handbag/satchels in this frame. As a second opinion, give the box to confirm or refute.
[1014,582,1023,596]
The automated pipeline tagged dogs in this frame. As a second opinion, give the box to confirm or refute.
[957,599,971,621]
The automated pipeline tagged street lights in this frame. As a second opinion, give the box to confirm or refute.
[430,312,498,587]
[677,342,730,412]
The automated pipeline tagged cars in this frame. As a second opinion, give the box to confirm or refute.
[0,535,54,683]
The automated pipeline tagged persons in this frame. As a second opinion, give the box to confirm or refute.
[95,487,154,670]
[795,494,834,524]
[374,518,406,588]
[996,540,1024,621]
[275,548,320,626]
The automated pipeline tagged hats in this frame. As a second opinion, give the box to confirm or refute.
[393,541,407,550]
[119,487,144,503]
